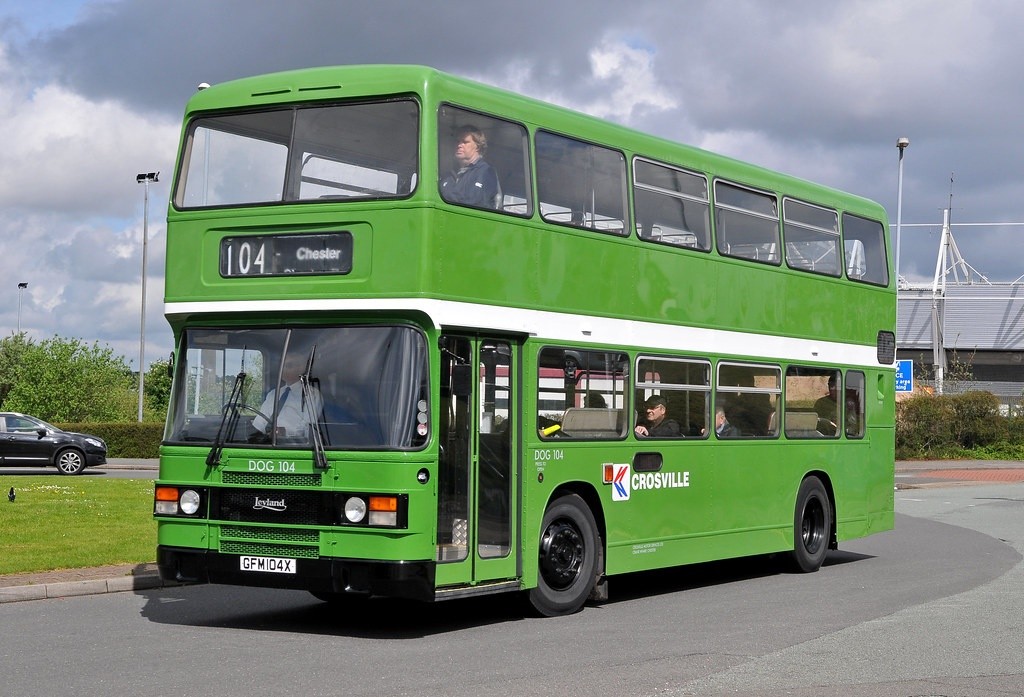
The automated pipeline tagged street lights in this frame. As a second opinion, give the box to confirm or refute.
[136,172,159,423]
[894,136,909,277]
[16,282,28,338]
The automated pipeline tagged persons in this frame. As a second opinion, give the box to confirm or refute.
[583,393,608,408]
[698,406,739,437]
[635,395,680,437]
[439,124,498,209]
[10,419,21,432]
[814,376,857,435]
[733,368,773,436]
[252,351,323,443]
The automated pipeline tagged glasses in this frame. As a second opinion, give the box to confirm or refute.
[284,362,299,369]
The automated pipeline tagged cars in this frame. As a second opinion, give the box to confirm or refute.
[0,412,108,476]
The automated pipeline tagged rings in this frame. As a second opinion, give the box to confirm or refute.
[276,431,278,434]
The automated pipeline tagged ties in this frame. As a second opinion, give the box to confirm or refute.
[265,387,291,433]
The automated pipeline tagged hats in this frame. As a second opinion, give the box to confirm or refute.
[643,394,669,407]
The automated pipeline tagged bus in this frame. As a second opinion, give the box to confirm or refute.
[153,63,899,620]
